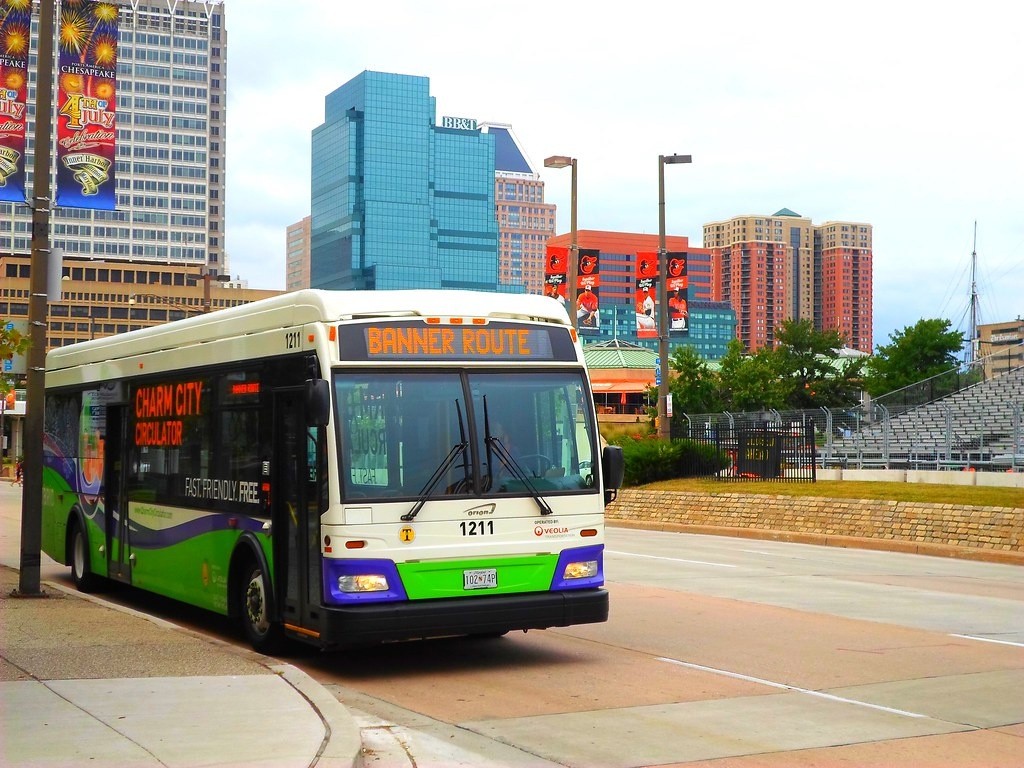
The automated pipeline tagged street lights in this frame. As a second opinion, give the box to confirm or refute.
[544,155,579,330]
[657,152,694,439]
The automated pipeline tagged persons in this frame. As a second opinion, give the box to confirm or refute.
[478,410,533,478]
[11,461,24,487]
[3,449,8,457]
[636,417,640,422]
[635,288,656,330]
[669,287,689,328]
[548,282,566,307]
[577,286,599,327]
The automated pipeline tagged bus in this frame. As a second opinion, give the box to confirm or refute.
[41,287,627,657]
